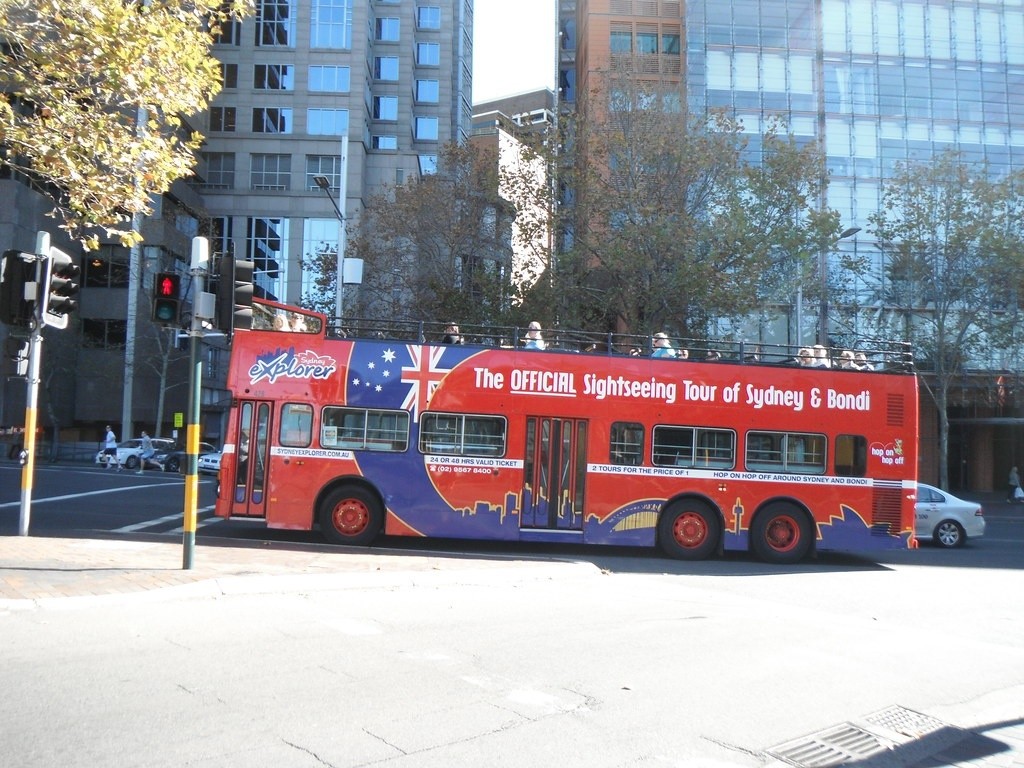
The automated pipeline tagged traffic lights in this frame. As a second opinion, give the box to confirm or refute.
[217,242,254,345]
[152,271,182,324]
[43,246,80,329]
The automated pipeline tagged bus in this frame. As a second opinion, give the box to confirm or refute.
[213,297,920,564]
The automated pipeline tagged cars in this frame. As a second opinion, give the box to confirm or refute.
[153,440,222,472]
[199,452,223,476]
[95,438,176,469]
[914,482,987,550]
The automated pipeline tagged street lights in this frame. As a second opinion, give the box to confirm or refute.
[312,174,346,338]
[795,226,863,353]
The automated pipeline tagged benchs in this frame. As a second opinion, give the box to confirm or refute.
[585,344,799,365]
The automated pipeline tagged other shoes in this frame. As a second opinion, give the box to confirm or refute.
[1020,500,1024,502]
[105,465,112,470]
[1007,499,1012,503]
[117,467,123,472]
[136,471,144,474]
[161,464,165,472]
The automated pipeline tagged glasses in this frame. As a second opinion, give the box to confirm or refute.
[106,427,111,429]
[655,338,659,343]
[840,356,846,357]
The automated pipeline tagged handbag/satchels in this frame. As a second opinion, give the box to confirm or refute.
[1015,486,1024,498]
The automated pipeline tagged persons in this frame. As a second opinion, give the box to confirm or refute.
[841,351,874,371]
[442,322,459,344]
[798,345,829,368]
[136,431,165,474]
[630,347,642,356]
[275,313,306,331]
[1007,466,1024,503]
[525,322,544,349]
[707,351,720,360]
[102,425,123,472]
[652,333,688,358]
